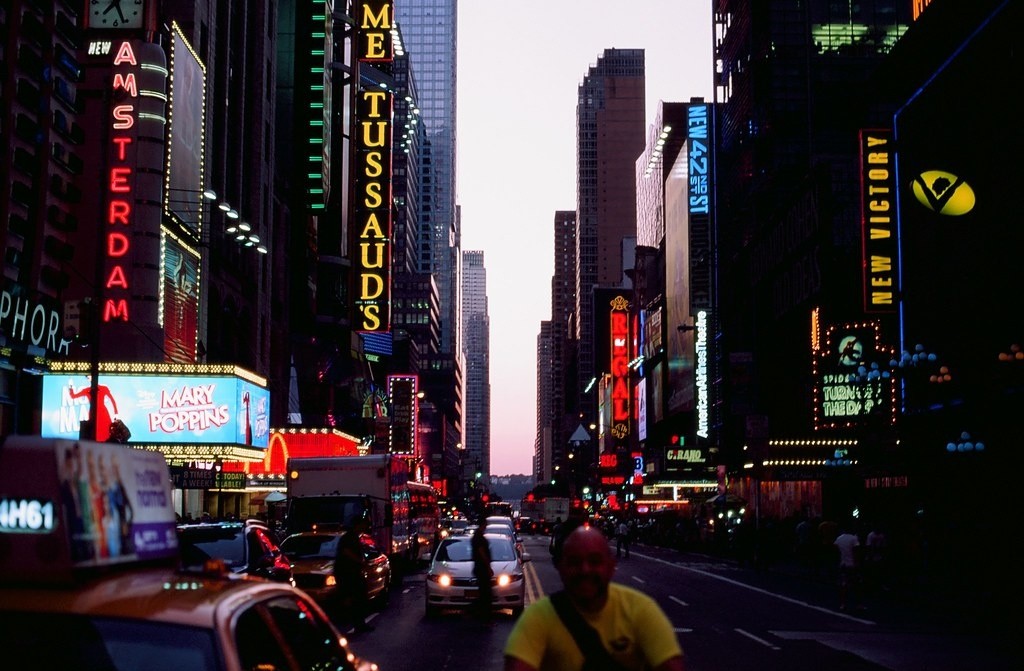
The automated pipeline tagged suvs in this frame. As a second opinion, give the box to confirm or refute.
[178,518,296,590]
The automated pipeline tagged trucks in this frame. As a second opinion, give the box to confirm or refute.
[288,454,417,587]
[522,498,570,528]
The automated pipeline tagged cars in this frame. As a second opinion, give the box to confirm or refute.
[424,516,531,618]
[0,577,382,671]
[280,531,391,611]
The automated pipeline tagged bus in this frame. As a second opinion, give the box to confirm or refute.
[407,482,442,566]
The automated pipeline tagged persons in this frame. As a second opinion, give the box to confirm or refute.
[459,517,497,627]
[504,526,688,671]
[549,517,563,561]
[513,516,548,535]
[730,515,928,634]
[590,518,697,557]
[68,375,118,442]
[328,514,373,635]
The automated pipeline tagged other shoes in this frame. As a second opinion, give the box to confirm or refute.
[355,622,376,633]
[482,619,498,627]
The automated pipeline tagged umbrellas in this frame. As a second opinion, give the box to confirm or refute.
[706,487,748,512]
[706,504,747,555]
